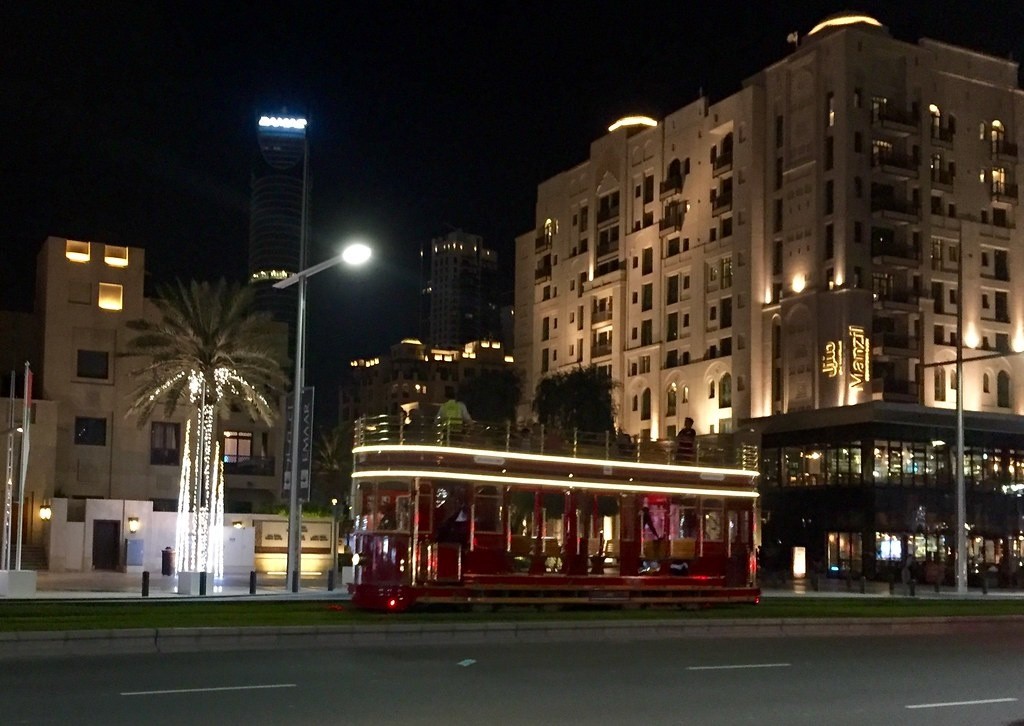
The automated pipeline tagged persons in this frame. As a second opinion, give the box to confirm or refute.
[510,408,572,452]
[677,417,697,462]
[618,434,637,457]
[377,500,396,530]
[390,390,478,455]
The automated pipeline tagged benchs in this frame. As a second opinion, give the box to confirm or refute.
[504,533,697,575]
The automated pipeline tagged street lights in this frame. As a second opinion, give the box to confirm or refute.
[269,242,374,596]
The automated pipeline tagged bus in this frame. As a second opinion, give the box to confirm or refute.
[348,410,765,616]
